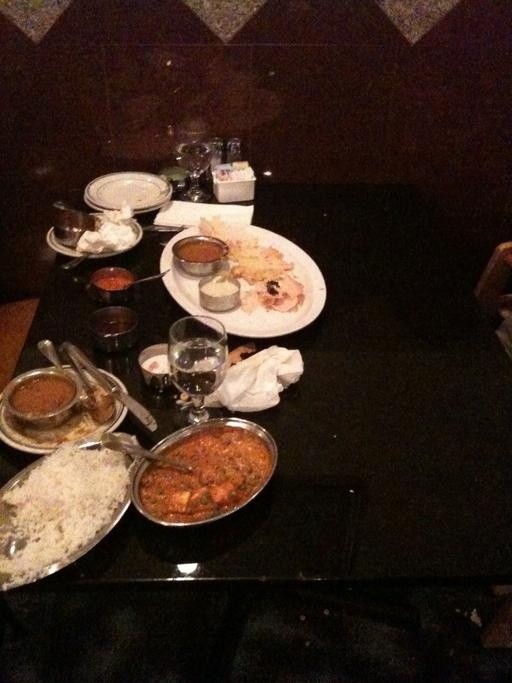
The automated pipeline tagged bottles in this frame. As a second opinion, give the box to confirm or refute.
[159,222,328,340]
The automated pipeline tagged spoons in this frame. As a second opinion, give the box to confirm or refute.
[56,343,117,424]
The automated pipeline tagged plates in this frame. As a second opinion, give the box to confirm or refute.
[1,429,143,609]
[46,212,144,260]
[1,364,128,455]
[83,171,174,215]
[124,415,277,527]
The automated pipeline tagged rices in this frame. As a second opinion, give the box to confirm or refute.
[1,443,131,591]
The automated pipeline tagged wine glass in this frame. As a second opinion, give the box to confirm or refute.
[173,141,215,203]
[167,316,230,429]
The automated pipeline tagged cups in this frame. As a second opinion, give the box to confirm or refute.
[85,264,140,355]
[52,209,98,248]
[138,342,169,391]
[207,136,242,166]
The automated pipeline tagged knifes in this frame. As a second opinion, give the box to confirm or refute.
[63,342,159,433]
